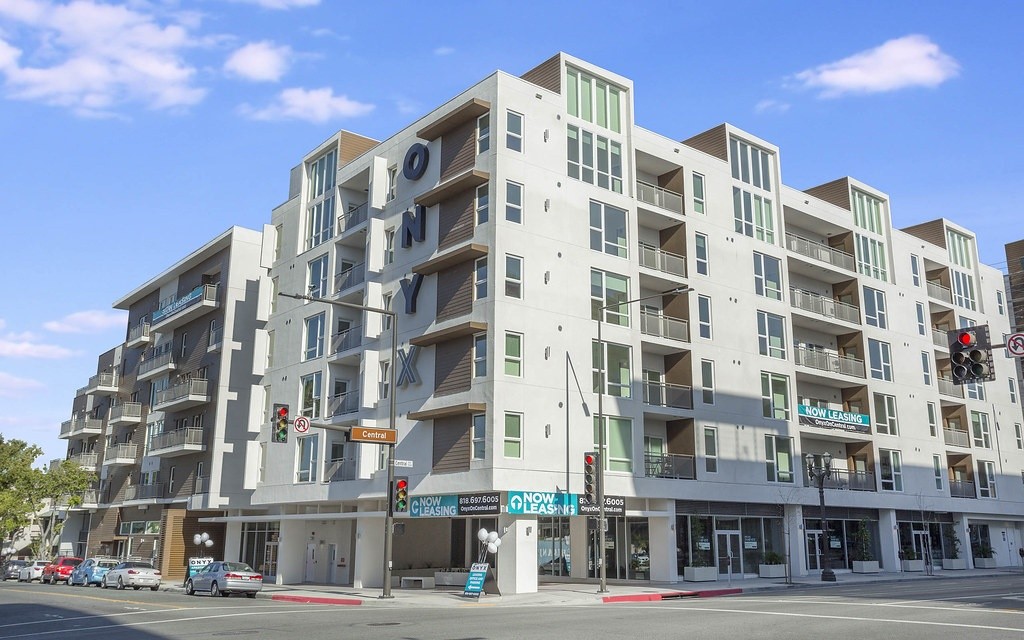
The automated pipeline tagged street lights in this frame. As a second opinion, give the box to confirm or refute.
[278,292,398,598]
[598,287,695,593]
[805,452,837,582]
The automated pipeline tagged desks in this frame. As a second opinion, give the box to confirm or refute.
[649,461,663,477]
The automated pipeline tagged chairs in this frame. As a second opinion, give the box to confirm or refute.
[645,459,679,479]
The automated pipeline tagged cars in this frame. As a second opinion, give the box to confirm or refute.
[18,560,52,583]
[68,558,121,586]
[101,562,162,591]
[185,561,262,598]
[0,560,30,581]
[632,553,649,566]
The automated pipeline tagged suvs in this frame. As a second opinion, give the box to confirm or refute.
[40,557,84,585]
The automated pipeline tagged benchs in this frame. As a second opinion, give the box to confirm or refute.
[401,577,435,589]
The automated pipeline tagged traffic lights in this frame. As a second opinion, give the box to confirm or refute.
[948,325,995,385]
[272,403,289,443]
[393,476,409,513]
[585,452,599,508]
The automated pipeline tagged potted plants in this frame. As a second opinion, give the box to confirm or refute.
[758,552,788,578]
[435,569,470,586]
[898,548,924,572]
[852,515,880,573]
[974,544,998,568]
[683,560,718,582]
[942,525,967,570]
